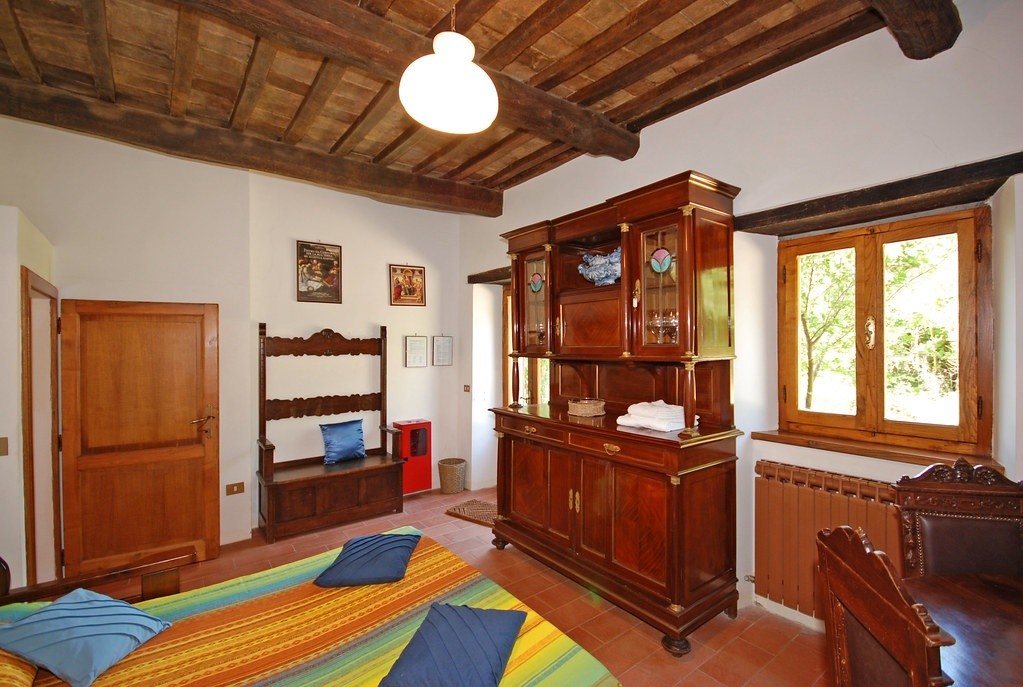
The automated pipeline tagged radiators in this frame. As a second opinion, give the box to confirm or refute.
[754,458,903,622]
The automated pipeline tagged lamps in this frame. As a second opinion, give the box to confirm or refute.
[397,0,500,135]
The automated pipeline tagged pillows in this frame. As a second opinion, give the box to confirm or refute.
[319,419,368,465]
[379,601,527,687]
[313,534,421,586]
[0,587,172,687]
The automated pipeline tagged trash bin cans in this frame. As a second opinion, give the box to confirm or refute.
[437,458,466,494]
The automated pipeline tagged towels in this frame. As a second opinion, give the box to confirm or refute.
[616,399,700,432]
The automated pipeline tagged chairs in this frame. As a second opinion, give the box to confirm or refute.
[810,523,958,687]
[888,456,1023,583]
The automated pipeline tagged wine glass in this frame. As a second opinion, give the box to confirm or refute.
[536,321,546,345]
[647,307,676,344]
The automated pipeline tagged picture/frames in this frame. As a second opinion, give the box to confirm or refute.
[389,264,426,306]
[296,240,343,304]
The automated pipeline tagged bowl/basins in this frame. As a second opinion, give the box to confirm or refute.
[567,400,605,416]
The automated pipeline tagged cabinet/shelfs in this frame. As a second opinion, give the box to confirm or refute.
[491,171,742,659]
[259,322,406,543]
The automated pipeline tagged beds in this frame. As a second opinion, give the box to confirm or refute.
[0,524,624,687]
